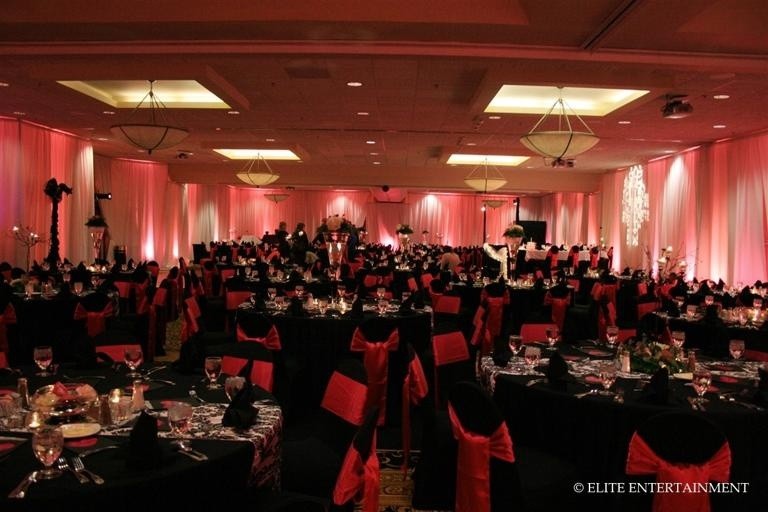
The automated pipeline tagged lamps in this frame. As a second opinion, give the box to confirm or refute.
[520,86,601,169]
[462,158,508,199]
[481,194,505,212]
[108,81,191,157]
[235,150,280,190]
[262,189,291,205]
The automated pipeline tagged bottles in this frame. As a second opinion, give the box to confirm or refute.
[17,377,29,411]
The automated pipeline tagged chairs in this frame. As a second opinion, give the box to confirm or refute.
[1,239,768,511]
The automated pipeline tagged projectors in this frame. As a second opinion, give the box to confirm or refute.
[661,100,693,121]
[551,159,576,171]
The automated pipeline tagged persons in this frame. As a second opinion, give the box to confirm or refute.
[276,221,289,256]
[440,245,462,274]
[345,228,366,261]
[292,222,309,267]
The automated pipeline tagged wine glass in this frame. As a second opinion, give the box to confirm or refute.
[245,268,251,278]
[26,274,99,300]
[30,350,246,481]
[510,327,745,404]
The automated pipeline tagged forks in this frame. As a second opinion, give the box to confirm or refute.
[57,457,104,487]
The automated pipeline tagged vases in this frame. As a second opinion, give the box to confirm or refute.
[398,233,409,252]
[507,237,523,271]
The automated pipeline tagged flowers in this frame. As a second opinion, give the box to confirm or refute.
[501,224,527,241]
[316,217,357,235]
[395,222,416,234]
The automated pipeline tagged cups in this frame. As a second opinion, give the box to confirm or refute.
[250,285,412,316]
[676,296,763,327]
